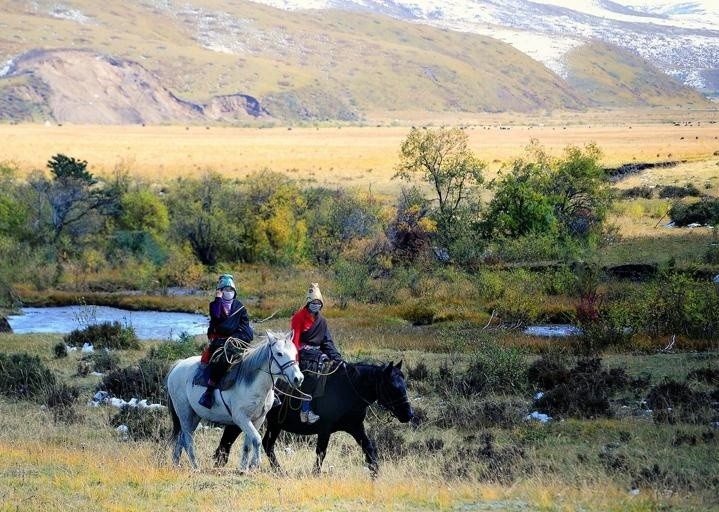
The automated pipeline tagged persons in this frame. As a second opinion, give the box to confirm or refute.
[197,274,253,410]
[292,284,342,424]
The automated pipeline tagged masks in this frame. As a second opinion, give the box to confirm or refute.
[222,290,235,301]
[309,303,321,313]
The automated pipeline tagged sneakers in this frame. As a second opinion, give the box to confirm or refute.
[300,410,320,423]
[198,391,216,409]
[272,396,279,407]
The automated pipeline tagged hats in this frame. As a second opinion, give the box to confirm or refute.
[216,274,237,295]
[305,281,324,308]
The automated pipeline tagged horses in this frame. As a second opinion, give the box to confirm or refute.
[211,359,414,482]
[164,330,305,472]
